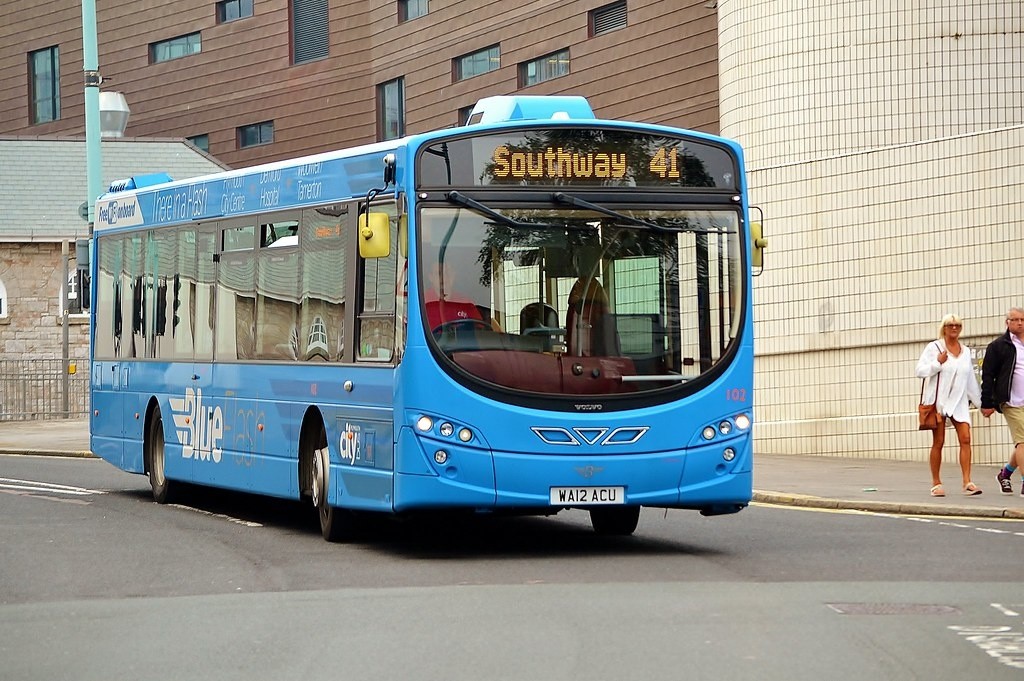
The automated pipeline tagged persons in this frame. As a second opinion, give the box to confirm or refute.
[424,256,487,336]
[915,314,994,496]
[981,307,1024,497]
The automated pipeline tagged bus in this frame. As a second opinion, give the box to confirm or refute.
[88,93,769,543]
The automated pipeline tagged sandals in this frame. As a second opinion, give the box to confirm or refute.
[961,483,983,496]
[931,484,945,497]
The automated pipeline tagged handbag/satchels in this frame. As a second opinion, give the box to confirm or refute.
[918,404,937,431]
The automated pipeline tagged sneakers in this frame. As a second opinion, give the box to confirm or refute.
[996,468,1014,495]
[1020,480,1024,498]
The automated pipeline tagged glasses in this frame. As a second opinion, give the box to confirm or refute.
[1008,318,1024,322]
[944,324,961,328]
